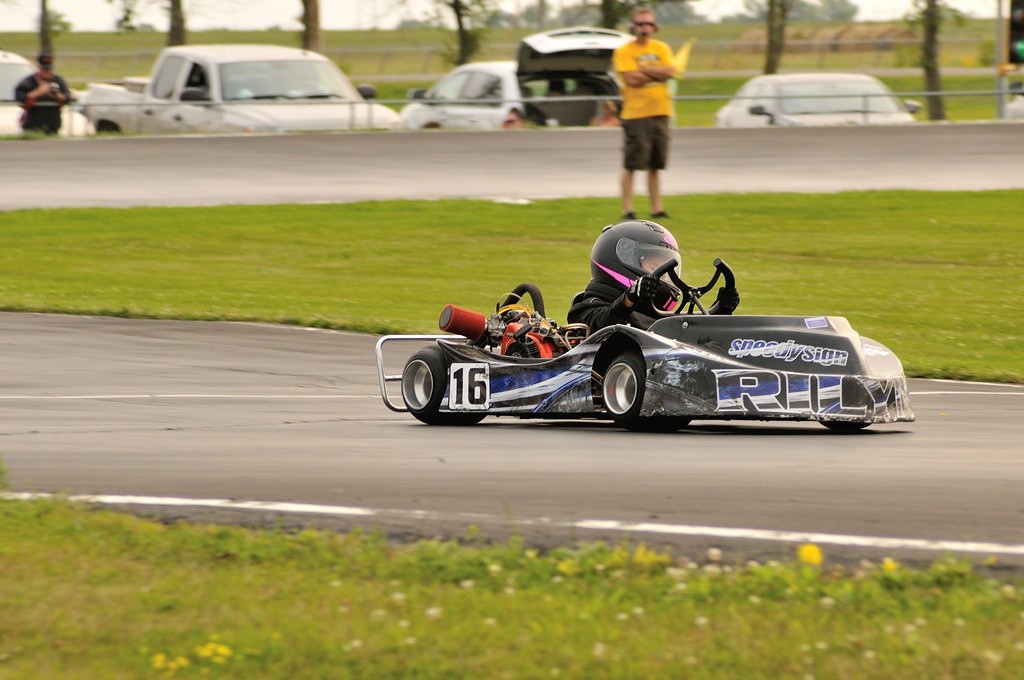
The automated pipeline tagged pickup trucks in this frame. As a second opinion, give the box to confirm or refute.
[83,41,405,140]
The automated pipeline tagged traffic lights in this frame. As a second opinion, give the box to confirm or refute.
[1007,0,1024,66]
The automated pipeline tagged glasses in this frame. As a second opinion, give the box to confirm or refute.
[504,117,523,126]
[631,21,655,27]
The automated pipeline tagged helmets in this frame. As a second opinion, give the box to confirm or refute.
[590,221,681,266]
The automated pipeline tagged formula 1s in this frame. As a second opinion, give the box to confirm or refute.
[373,256,917,438]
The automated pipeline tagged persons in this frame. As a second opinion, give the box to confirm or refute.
[15,49,72,139]
[612,5,680,225]
[566,219,739,346]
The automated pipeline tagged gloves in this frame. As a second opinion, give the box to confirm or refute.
[714,287,743,313]
[625,274,662,304]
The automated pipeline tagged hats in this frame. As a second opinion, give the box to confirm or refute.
[37,53,54,70]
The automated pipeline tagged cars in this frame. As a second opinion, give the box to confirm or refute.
[1002,82,1018,122]
[401,22,638,128]
[1,52,94,142]
[712,71,923,131]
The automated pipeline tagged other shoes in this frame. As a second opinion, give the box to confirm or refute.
[651,211,672,220]
[621,211,637,223]
